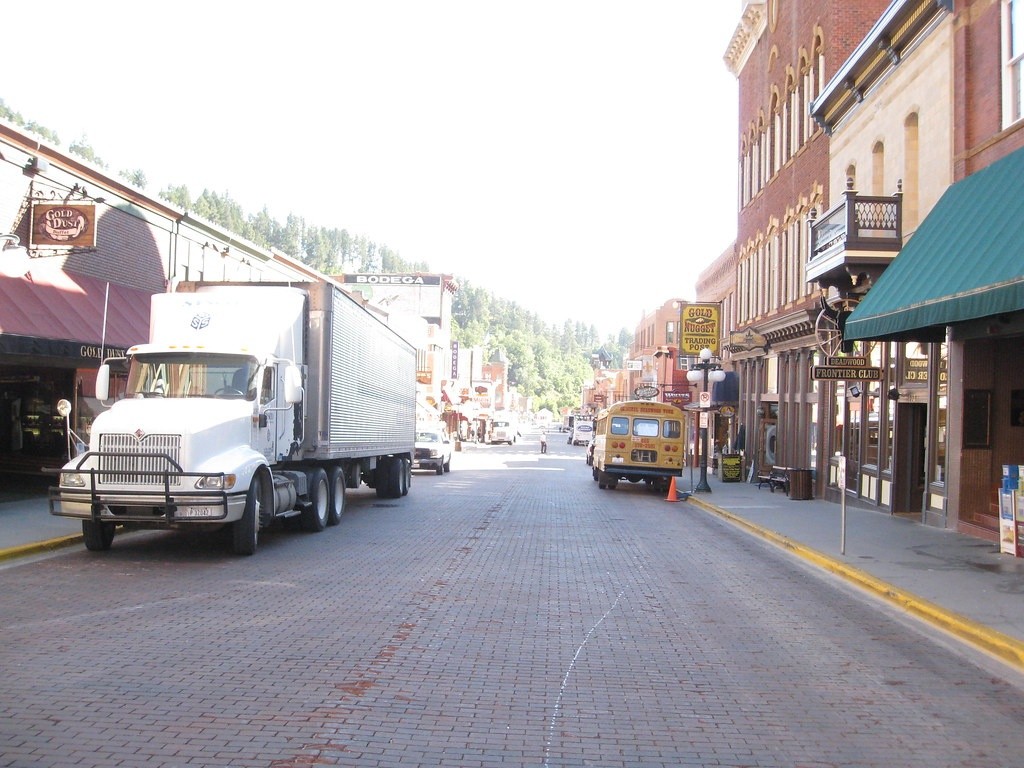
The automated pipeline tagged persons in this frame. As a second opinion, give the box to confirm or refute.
[541,432,546,453]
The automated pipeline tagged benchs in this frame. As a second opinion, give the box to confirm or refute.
[0,451,67,477]
[757,465,801,497]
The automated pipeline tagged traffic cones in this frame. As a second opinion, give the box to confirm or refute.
[664,475,681,503]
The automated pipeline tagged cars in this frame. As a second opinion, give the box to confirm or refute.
[586,439,595,466]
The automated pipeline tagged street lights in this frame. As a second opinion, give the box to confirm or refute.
[453,396,463,453]
[686,348,727,495]
[472,402,481,445]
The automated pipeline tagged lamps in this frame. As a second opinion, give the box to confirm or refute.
[887,387,908,401]
[757,402,765,416]
[849,386,862,398]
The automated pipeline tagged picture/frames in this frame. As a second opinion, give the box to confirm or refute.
[962,389,992,449]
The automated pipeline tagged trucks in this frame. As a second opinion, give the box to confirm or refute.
[572,420,593,446]
[491,415,518,446]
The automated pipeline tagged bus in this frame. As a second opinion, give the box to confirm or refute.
[592,400,686,494]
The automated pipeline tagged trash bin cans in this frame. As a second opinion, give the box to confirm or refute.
[789,469,816,500]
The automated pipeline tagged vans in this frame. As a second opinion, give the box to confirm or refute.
[410,420,452,476]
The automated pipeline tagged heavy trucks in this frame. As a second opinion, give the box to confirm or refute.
[48,280,419,559]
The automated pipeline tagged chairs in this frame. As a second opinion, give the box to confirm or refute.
[233,369,247,395]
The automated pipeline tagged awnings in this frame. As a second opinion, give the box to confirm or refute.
[0,260,156,368]
[443,390,459,406]
[844,148,1024,343]
